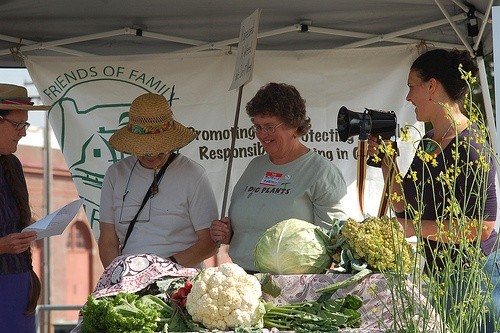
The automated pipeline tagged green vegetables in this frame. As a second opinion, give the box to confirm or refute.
[263,288,363,333]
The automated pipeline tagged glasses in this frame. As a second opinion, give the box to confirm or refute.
[0,115,30,131]
[250,122,284,135]
[118,191,153,223]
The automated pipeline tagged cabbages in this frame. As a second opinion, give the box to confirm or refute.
[254,218,333,275]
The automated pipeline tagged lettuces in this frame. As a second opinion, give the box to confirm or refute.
[80,289,164,333]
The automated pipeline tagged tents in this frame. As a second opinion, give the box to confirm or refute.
[0,0,500,333]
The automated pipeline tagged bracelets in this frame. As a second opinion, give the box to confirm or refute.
[169,256,178,264]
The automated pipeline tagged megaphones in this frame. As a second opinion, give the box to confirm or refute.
[337,106,397,168]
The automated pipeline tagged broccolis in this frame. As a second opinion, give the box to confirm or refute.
[185,262,265,330]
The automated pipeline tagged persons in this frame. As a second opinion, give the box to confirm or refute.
[0,84,53,333]
[99,93,219,269]
[209,83,351,276]
[367,50,500,333]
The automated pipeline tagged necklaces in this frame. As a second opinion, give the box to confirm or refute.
[423,115,462,152]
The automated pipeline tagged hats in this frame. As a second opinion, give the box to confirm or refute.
[109,93,197,157]
[0,83,52,111]
